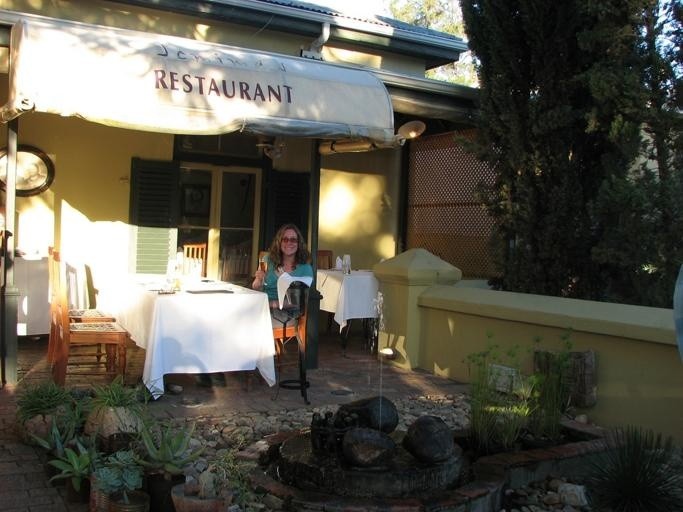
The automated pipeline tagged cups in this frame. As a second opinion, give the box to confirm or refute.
[341,254,351,276]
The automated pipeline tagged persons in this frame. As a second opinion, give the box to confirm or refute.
[195,223,313,386]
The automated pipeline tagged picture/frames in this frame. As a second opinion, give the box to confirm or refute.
[0,145,57,196]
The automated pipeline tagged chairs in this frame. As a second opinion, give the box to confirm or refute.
[314,249,333,271]
[45,249,130,390]
[43,244,117,374]
[266,286,310,371]
[181,241,208,277]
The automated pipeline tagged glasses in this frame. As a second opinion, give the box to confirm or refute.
[282,236,299,244]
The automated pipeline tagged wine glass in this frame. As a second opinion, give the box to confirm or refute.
[258,260,268,285]
